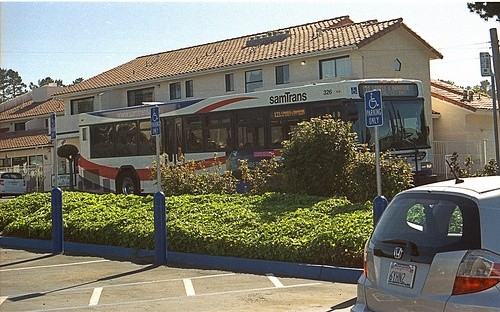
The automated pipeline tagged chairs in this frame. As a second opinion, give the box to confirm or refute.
[207,141,217,151]
[424,214,448,238]
[190,140,201,151]
[3,175,8,178]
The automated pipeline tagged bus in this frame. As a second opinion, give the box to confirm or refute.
[77,78,433,198]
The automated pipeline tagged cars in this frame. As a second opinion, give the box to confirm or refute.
[350,175,500,312]
[0,172,26,198]
